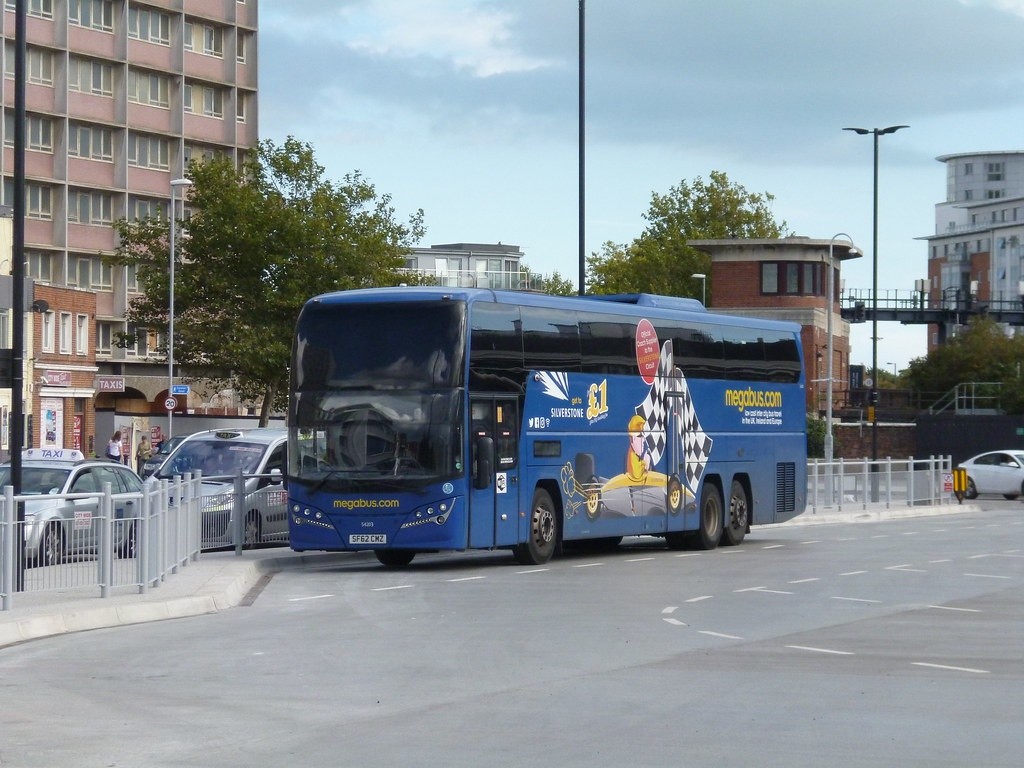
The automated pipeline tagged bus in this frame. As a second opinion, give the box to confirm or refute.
[284,289,808,566]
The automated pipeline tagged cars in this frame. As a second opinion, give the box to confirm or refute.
[960,450,1023,500]
[145,426,326,550]
[139,433,234,481]
[0,445,146,567]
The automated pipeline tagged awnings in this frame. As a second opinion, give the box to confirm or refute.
[93,373,265,403]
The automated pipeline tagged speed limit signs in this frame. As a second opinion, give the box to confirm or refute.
[165,397,178,410]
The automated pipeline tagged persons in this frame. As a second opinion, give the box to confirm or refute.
[154,433,169,454]
[136,436,152,475]
[105,432,125,464]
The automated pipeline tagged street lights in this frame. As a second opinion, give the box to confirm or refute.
[824,232,858,508]
[168,175,195,439]
[844,125,910,501]
[691,273,706,307]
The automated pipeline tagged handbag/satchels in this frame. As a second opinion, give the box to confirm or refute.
[105,446,110,458]
